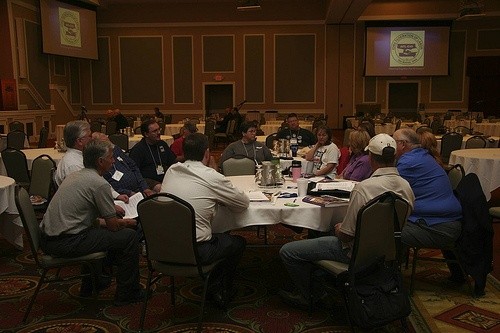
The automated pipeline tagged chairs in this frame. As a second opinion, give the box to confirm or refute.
[0,110,500,333]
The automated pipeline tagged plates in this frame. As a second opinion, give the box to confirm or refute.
[257,184,284,188]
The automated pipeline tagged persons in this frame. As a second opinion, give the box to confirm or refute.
[279,133,416,309]
[40,105,463,308]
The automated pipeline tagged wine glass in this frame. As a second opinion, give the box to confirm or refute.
[273,138,290,157]
[254,161,282,186]
[446,128,462,136]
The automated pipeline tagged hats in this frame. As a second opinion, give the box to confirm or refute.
[363,133,398,156]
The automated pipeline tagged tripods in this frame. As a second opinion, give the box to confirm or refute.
[79,106,89,123]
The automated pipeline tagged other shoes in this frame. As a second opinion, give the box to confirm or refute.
[213,291,230,311]
[278,290,312,310]
[311,287,329,305]
[113,282,153,306]
[79,274,112,296]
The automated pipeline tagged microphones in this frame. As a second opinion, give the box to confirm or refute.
[238,100,247,107]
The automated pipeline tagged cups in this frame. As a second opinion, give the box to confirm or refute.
[297,176,309,197]
[291,144,297,157]
[291,159,302,182]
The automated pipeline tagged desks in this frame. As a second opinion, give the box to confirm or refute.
[165,124,205,135]
[128,134,174,149]
[10,148,65,171]
[278,156,314,176]
[434,135,500,157]
[448,148,500,202]
[0,175,23,248]
[374,123,420,136]
[212,175,361,246]
[260,125,313,135]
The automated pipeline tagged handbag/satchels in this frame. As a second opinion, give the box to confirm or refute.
[344,269,412,331]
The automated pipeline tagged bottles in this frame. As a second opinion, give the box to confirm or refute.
[131,128,134,136]
